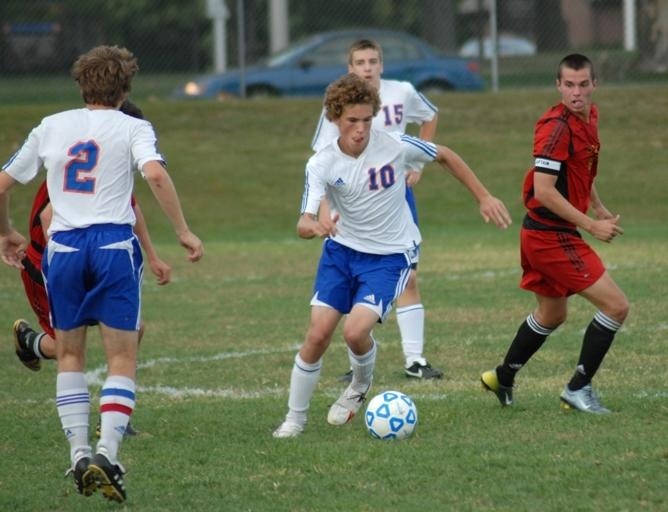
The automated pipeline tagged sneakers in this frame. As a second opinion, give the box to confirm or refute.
[13,319,42,372]
[90,451,127,502]
[481,365,514,406]
[71,452,95,496]
[340,365,358,383]
[327,378,374,426]
[404,358,445,380]
[97,406,155,442]
[274,408,309,440]
[557,385,613,417]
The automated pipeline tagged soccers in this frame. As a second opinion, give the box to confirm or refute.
[364,390,419,439]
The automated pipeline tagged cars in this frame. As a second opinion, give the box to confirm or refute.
[169,27,484,101]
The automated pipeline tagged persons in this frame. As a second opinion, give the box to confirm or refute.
[481,55,631,417]
[312,38,444,380]
[271,74,512,438]
[0,47,202,503]
[12,100,173,437]
